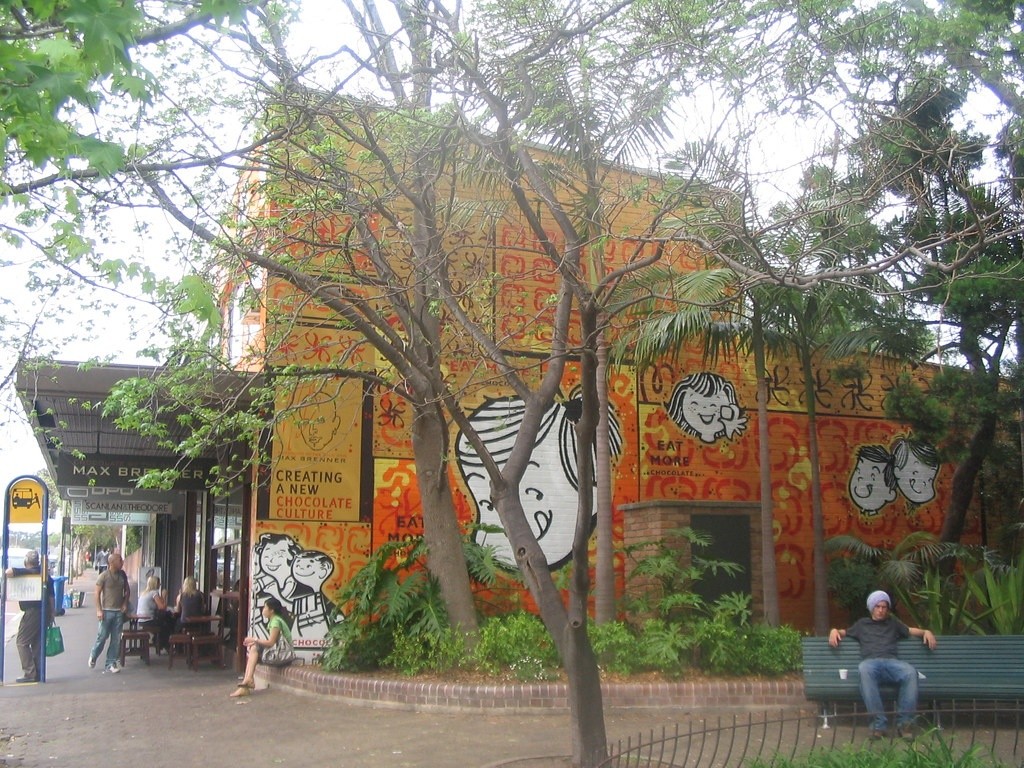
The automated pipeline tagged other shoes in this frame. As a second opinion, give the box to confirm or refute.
[230,688,250,697]
[869,729,886,739]
[16,676,38,682]
[897,728,914,742]
[238,680,254,688]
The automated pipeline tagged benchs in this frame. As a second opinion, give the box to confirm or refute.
[802,634,1024,730]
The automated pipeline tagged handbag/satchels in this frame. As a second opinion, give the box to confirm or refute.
[44,620,64,656]
[261,620,296,666]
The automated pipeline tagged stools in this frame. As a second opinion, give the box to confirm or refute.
[121,624,225,672]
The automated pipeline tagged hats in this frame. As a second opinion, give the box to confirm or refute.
[866,590,891,613]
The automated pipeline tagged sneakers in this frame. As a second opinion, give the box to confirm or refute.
[89,656,96,668]
[104,665,120,673]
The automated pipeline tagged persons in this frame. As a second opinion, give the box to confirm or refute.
[829,591,937,742]
[172,577,203,651]
[95,545,109,574]
[88,554,130,673]
[5,551,55,683]
[137,576,175,654]
[230,598,294,697]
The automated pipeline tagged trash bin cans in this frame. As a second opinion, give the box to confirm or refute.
[51,576,69,616]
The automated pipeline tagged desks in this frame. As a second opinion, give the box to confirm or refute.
[183,615,222,635]
[121,615,152,647]
